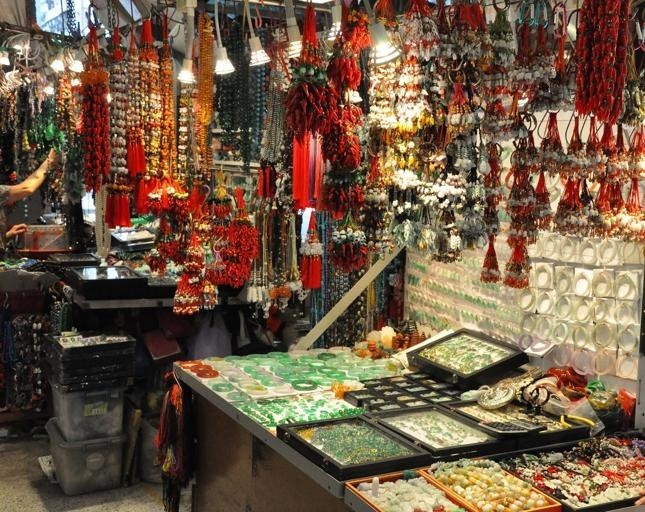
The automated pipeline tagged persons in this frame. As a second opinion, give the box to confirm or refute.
[0,148,57,254]
[0,267,73,299]
[0,290,18,412]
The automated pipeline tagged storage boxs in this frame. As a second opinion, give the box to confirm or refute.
[48,377,132,442]
[45,417,122,497]
[52,331,136,392]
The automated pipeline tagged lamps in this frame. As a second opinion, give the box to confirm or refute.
[0,32,31,67]
[49,35,87,76]
[172,0,404,86]
